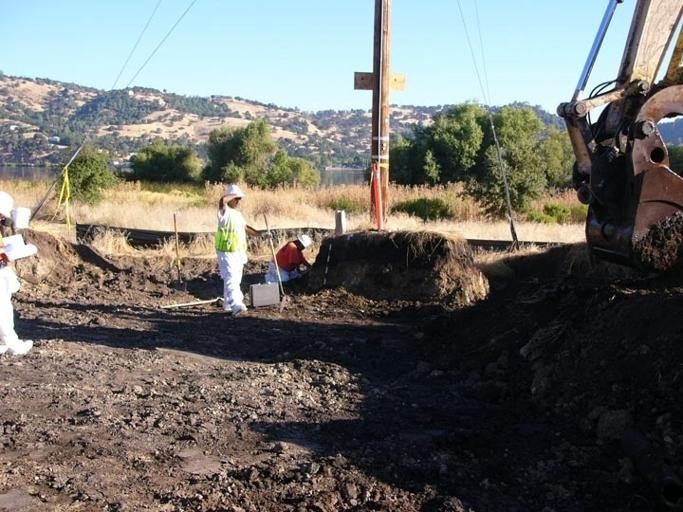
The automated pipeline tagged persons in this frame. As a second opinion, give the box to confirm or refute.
[1,192,32,355]
[264,232,314,282]
[213,183,269,319]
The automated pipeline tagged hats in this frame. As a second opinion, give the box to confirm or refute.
[226,185,245,197]
[0,193,13,218]
[298,235,311,248]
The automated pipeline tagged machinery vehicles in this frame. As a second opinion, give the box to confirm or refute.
[556,2,683,288]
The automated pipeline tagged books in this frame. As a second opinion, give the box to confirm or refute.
[1,233,38,260]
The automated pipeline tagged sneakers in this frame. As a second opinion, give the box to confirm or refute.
[232,303,246,317]
[20,339,34,356]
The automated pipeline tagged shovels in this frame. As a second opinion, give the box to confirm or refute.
[174,214,187,293]
[159,297,225,310]
[264,210,291,314]
